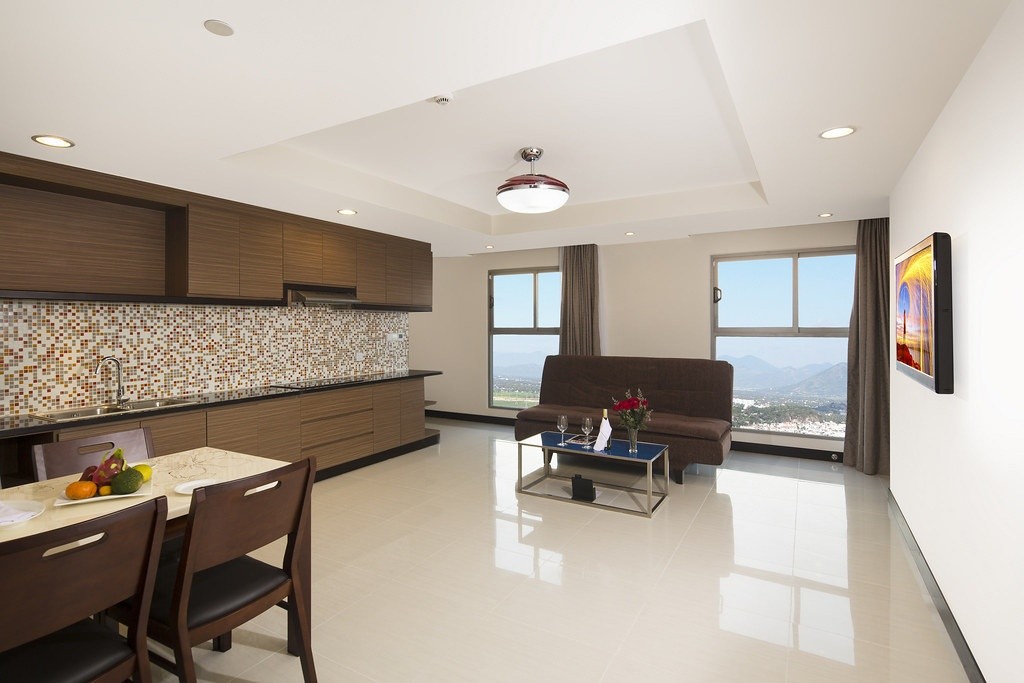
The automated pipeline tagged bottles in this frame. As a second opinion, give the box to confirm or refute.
[602,409,610,450]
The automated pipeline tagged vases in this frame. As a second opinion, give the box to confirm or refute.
[627,428,639,453]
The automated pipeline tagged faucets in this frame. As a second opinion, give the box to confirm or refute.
[94,356,130,406]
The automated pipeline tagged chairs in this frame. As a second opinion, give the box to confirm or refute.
[32,427,155,483]
[0,495,168,683]
[103,456,318,683]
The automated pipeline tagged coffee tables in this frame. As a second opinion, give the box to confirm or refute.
[517,430,669,516]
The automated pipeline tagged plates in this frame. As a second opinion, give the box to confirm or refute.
[53,461,153,508]
[174,479,222,494]
[0,500,46,527]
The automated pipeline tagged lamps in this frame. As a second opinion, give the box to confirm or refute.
[496,147,569,214]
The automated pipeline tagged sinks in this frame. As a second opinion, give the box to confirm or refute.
[27,406,131,424]
[107,398,201,413]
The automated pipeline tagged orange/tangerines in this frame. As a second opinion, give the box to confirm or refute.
[66,480,97,500]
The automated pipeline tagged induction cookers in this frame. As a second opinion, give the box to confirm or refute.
[270,378,365,389]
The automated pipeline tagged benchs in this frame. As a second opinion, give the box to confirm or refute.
[514,355,733,485]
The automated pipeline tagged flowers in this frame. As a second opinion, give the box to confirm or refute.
[611,389,653,447]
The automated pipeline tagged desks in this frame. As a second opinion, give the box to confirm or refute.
[0,446,310,657]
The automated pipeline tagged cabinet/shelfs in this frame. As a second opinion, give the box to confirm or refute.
[55,369,443,470]
[186,204,433,310]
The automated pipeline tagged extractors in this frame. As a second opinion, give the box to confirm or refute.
[286,289,362,307]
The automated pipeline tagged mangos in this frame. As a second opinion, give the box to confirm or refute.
[133,464,153,482]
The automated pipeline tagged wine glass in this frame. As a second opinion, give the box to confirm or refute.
[581,417,593,449]
[557,415,568,446]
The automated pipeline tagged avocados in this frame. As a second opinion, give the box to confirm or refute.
[112,468,144,495]
